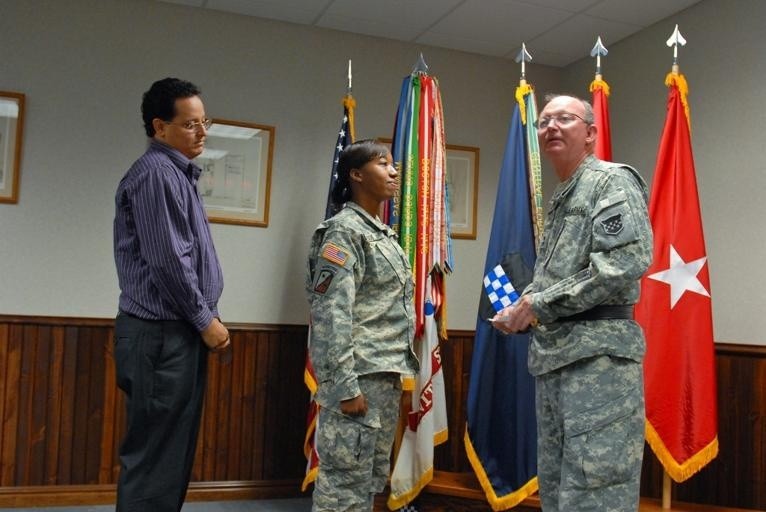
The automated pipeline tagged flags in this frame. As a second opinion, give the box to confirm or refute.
[492,95,654,512]
[463,79,543,512]
[383,74,454,512]
[633,72,719,480]
[301,95,357,493]
[590,74,613,161]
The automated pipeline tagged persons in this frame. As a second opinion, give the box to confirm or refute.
[304,138,420,512]
[113,78,231,512]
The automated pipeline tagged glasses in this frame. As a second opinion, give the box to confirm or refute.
[166,118,212,133]
[534,112,591,127]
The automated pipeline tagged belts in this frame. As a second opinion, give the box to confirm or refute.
[532,302,633,324]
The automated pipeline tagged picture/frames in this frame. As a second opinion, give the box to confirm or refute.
[0,89,25,206]
[189,117,276,229]
[374,137,480,240]
